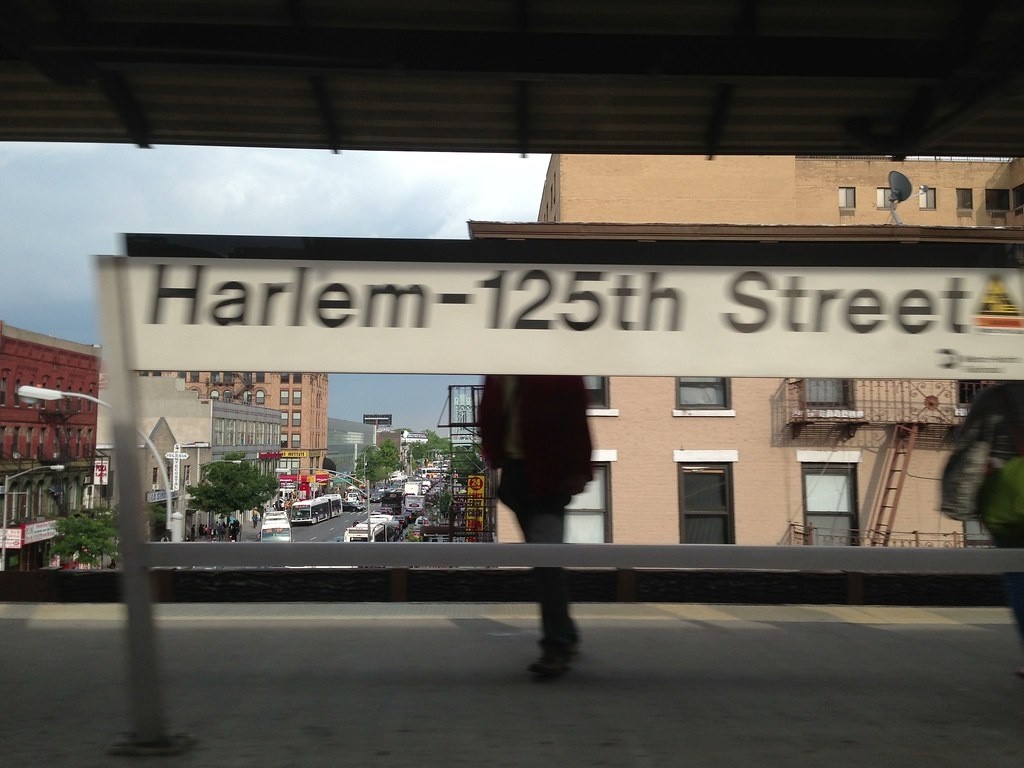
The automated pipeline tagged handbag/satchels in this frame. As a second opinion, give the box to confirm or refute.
[939,413,1005,521]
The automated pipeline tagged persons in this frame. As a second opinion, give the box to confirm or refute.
[257,532,260,541]
[183,514,239,542]
[957,379,1024,674]
[110,559,116,569]
[353,519,361,527]
[252,514,258,528]
[477,375,594,677]
[72,562,78,570]
[161,534,169,542]
[93,565,97,569]
[265,505,274,512]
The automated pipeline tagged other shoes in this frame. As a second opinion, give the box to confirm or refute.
[526,644,575,679]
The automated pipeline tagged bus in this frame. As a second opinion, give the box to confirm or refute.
[343,515,395,542]
[382,470,409,515]
[261,511,291,542]
[290,493,343,526]
[418,468,448,478]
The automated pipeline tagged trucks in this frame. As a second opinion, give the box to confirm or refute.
[403,482,422,505]
[404,495,425,514]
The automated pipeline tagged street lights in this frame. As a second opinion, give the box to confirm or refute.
[17,385,184,543]
[1,465,64,572]
[410,446,419,475]
[275,468,371,543]
[364,445,381,482]
[183,460,241,540]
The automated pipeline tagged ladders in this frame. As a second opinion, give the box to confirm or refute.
[869,425,918,547]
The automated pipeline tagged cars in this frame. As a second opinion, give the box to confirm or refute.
[342,501,363,512]
[414,478,441,495]
[370,507,413,534]
[370,493,383,503]
